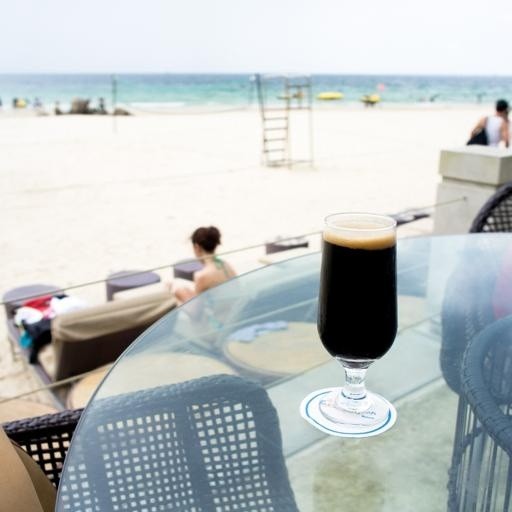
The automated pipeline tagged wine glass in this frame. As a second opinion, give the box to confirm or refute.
[316,211,399,426]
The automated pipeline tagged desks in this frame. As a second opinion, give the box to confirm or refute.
[53,230,512,512]
[0,208,432,318]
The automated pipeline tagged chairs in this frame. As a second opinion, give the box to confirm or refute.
[0,372,300,512]
[439,184,512,512]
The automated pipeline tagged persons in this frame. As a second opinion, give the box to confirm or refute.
[171,224,239,343]
[465,98,512,148]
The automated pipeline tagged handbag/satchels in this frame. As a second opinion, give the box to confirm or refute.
[468,128,488,144]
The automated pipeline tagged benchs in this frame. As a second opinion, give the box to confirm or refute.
[39,280,196,384]
